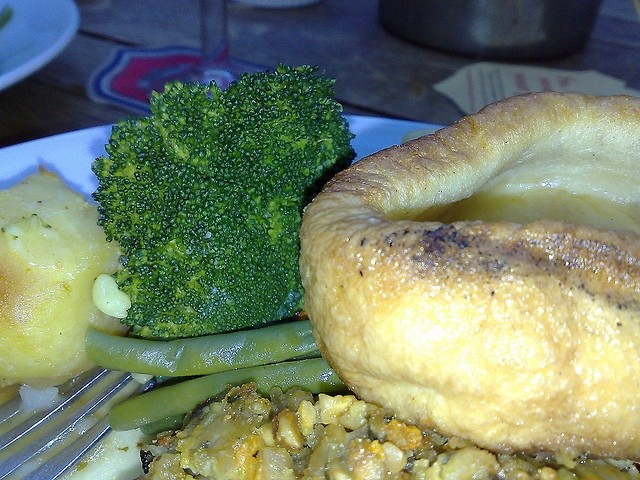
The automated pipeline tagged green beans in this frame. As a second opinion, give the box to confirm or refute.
[105,353,344,431]
[82,320,319,378]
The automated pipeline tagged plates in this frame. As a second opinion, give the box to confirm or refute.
[0,0,80,89]
[0,113,449,208]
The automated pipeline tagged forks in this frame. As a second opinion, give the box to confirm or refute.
[1,365,156,480]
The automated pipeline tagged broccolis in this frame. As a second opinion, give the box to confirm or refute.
[91,63,357,339]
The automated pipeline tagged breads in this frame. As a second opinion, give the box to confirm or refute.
[299,93,639,460]
